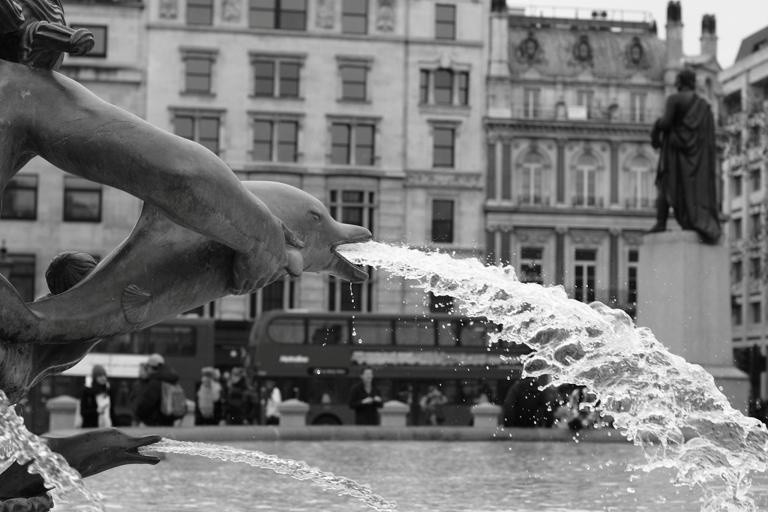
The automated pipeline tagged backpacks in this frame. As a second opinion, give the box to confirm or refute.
[159,381,187,418]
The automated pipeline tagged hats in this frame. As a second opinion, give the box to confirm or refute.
[147,352,166,368]
[93,365,106,376]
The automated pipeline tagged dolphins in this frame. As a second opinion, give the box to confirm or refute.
[0,428,163,501]
[0,180,374,404]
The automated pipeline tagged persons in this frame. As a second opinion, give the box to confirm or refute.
[348,365,384,424]
[128,353,282,426]
[648,69,723,244]
[80,364,118,428]
[0,1,305,399]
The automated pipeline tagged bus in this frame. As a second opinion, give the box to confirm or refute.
[234,306,594,429]
[76,315,252,404]
